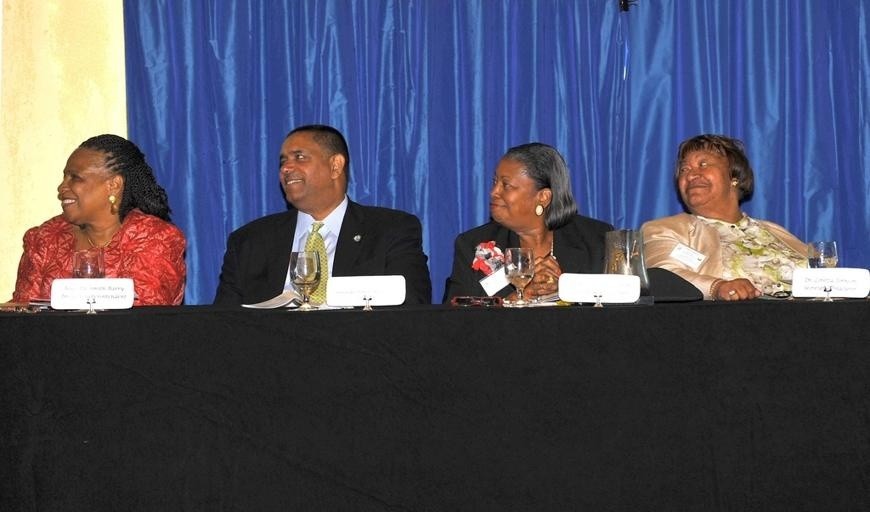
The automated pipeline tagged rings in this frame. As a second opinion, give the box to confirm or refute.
[728,289,738,296]
[546,275,555,285]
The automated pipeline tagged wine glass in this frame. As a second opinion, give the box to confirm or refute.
[287,252,325,312]
[807,240,843,303]
[503,247,536,307]
[67,247,111,316]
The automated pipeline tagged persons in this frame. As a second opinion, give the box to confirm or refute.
[630,134,824,304]
[8,134,187,309]
[210,123,432,308]
[444,141,621,306]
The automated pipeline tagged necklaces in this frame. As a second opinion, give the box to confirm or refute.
[83,231,113,249]
[547,228,556,257]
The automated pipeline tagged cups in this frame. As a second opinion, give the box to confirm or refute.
[603,228,651,299]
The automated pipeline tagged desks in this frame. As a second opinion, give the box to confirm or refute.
[0,297,870,511]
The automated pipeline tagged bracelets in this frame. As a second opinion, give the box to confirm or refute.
[709,277,725,298]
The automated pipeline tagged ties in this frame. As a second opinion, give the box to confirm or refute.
[304,222,328,306]
